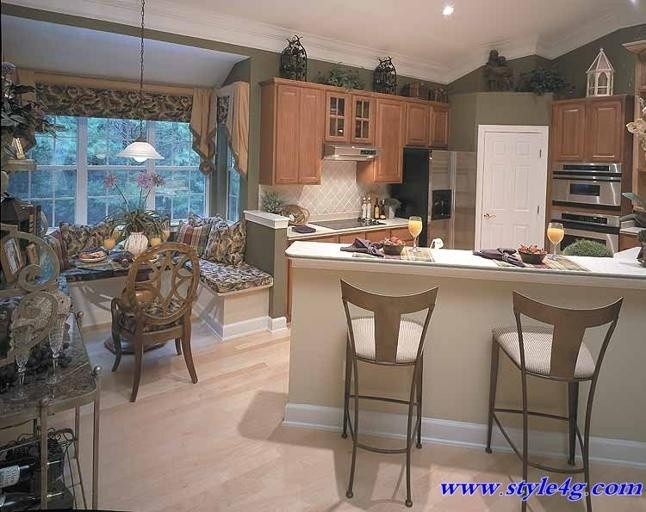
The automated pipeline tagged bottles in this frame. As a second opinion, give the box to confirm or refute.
[361,197,366,219]
[374,197,379,219]
[366,195,372,218]
[380,199,386,220]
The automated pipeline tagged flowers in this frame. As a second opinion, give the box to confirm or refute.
[101,172,167,241]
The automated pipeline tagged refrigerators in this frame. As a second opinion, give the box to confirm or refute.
[390,148,476,250]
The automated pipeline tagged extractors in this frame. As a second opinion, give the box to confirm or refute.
[320,143,381,163]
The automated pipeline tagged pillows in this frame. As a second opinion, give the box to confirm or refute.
[176,213,248,267]
[43,230,71,273]
[59,221,106,255]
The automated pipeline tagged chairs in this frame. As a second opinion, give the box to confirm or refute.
[109,240,202,402]
[338,278,441,508]
[485,288,624,511]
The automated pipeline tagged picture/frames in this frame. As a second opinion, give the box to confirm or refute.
[24,241,40,266]
[0,229,26,283]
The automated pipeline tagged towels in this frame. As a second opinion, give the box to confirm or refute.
[473,247,526,268]
[340,237,384,257]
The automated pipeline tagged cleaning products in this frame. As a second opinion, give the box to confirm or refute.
[362,194,371,219]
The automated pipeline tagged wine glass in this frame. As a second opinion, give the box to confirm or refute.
[547,222,564,260]
[150,234,162,249]
[103,236,115,259]
[408,216,422,253]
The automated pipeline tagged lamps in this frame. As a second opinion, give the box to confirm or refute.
[117,1,166,163]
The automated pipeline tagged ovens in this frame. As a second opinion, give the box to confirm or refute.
[550,161,622,258]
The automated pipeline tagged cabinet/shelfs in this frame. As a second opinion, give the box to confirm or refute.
[550,95,634,163]
[0,156,102,511]
[357,92,404,185]
[619,230,646,259]
[286,236,339,327]
[404,96,452,149]
[258,79,325,186]
[326,85,375,146]
[339,230,391,245]
[623,39,646,227]
[391,228,420,247]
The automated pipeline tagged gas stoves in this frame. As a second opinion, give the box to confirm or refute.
[307,218,389,231]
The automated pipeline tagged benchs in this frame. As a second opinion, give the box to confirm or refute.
[58,235,274,344]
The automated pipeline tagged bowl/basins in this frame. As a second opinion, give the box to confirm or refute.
[518,248,547,263]
[381,240,407,255]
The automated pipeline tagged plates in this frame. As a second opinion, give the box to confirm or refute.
[132,254,160,265]
[80,252,107,263]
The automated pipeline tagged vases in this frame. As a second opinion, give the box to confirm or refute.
[124,232,149,263]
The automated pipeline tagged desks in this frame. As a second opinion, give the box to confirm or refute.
[282,240,646,471]
